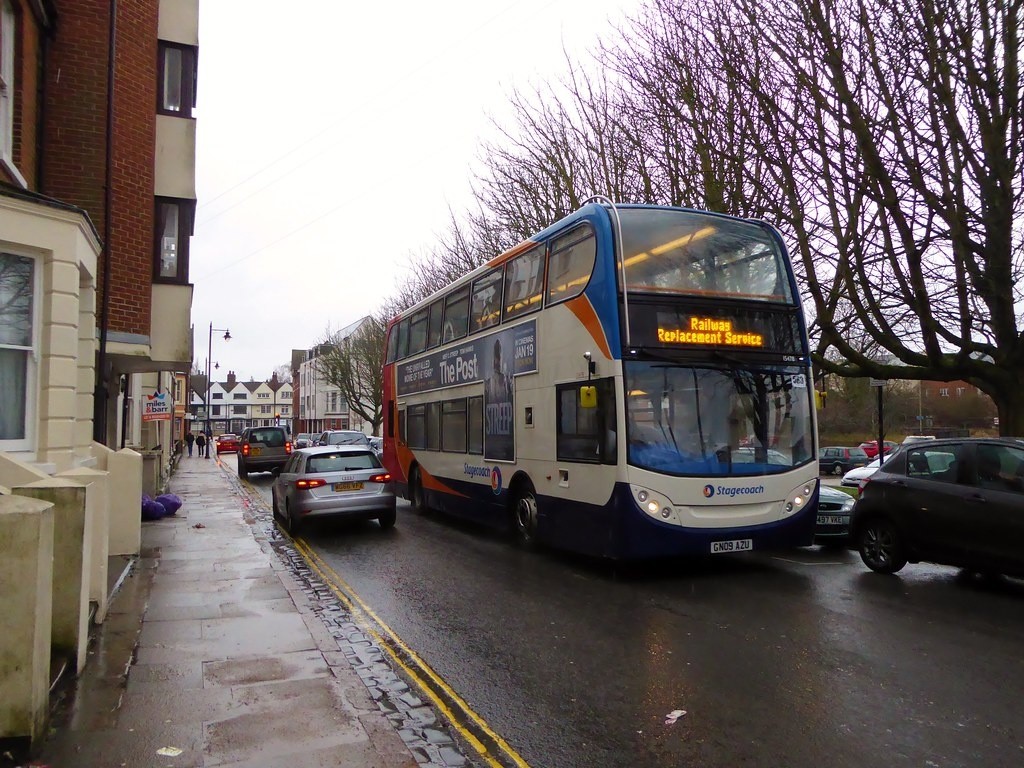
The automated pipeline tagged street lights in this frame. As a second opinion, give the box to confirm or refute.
[203,321,232,459]
[202,358,221,434]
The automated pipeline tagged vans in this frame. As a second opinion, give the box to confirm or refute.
[233,425,292,478]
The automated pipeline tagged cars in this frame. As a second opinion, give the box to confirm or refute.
[215,433,241,454]
[270,444,398,538]
[819,446,869,476]
[294,430,384,464]
[840,450,955,489]
[730,446,857,550]
[857,440,900,464]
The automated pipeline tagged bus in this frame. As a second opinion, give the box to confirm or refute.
[375,193,828,578]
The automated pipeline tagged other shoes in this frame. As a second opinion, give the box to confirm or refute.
[202,455,203,457]
[189,456,190,457]
[199,455,200,457]
[191,455,194,456]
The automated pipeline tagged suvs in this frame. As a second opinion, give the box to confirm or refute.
[851,434,1024,593]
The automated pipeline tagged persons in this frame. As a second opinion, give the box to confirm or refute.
[484,339,514,403]
[185,430,207,458]
